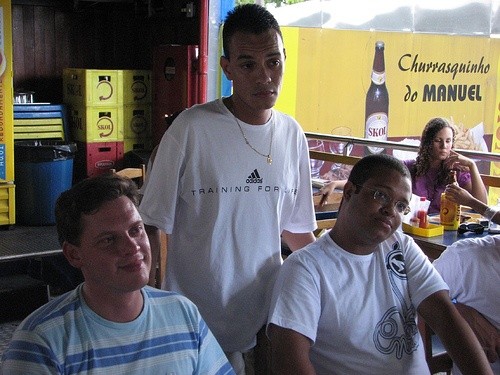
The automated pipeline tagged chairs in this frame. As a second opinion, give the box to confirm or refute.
[414,312,453,375]
[110,163,145,189]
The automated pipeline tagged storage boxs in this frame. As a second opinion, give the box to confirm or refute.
[63,66,150,179]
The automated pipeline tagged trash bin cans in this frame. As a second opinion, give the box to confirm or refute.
[15,138,80,227]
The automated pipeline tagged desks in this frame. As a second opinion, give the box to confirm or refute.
[402,213,500,260]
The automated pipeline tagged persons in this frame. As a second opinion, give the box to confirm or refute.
[138,3,318,375]
[2,173,235,375]
[313,118,488,216]
[266,155,495,375]
[432,231,500,375]
[444,183,500,224]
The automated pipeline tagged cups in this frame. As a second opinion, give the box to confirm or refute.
[440,192,461,230]
[12,88,38,104]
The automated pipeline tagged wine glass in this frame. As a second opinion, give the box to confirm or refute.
[306,139,325,183]
[329,127,354,178]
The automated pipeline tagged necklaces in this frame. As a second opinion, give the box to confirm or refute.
[223,97,274,165]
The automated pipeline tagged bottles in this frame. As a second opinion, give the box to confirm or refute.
[410,217,420,228]
[447,170,459,189]
[416,197,428,228]
[364,41,390,155]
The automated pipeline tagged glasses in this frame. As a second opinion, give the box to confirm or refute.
[358,184,411,214]
